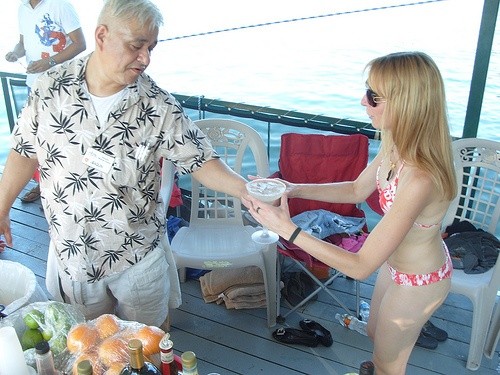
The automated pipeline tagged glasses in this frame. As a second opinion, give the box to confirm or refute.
[367,89,388,106]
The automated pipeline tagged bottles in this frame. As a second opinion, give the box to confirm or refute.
[75,360,94,375]
[34,341,62,375]
[335,313,369,337]
[120,338,162,375]
[181,351,199,375]
[359,300,370,322]
[158,333,183,375]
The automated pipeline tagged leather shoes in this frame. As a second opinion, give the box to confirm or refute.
[271,327,318,347]
[299,318,333,347]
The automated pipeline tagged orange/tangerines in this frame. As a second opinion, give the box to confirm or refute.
[67,314,165,375]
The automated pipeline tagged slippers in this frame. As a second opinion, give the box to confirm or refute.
[420,319,448,341]
[20,185,41,202]
[415,333,437,349]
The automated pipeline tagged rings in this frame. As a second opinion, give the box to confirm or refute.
[255,207,261,212]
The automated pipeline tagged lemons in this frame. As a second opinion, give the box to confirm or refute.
[20,303,74,354]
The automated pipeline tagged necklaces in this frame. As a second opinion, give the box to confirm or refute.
[387,145,403,181]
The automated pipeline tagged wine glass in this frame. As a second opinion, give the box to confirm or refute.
[245,178,287,244]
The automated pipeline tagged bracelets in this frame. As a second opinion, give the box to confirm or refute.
[288,227,302,244]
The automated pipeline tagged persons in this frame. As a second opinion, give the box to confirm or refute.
[5,0,86,211]
[0,0,283,330]
[246,52,458,375]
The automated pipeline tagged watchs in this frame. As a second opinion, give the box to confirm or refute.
[48,57,57,66]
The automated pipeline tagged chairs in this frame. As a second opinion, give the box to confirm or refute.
[441,137,500,370]
[157,118,276,328]
[266,133,369,324]
